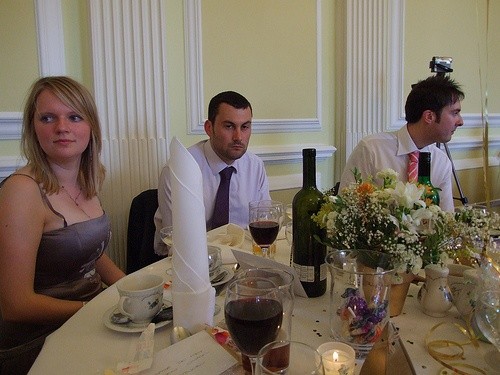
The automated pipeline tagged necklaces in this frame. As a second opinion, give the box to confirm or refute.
[55,176,83,207]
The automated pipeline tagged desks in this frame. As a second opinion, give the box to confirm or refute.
[28,225,500,375]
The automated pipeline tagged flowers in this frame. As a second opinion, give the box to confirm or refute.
[309,166,500,284]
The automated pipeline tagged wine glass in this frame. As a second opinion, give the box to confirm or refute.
[224,277,283,375]
[159,226,173,275]
[250,199,292,258]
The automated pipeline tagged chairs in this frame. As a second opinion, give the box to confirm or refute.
[127,189,158,275]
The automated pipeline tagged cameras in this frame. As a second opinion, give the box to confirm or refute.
[430,55,454,72]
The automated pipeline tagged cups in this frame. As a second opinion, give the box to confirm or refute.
[254,340,325,375]
[237,268,294,373]
[117,272,164,324]
[208,245,223,280]
[325,249,401,355]
[318,342,355,375]
[474,238,500,351]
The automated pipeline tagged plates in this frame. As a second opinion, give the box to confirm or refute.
[103,299,172,332]
[211,264,235,286]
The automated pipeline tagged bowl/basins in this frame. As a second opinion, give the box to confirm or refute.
[446,264,473,297]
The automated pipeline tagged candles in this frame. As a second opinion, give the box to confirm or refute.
[321,351,355,374]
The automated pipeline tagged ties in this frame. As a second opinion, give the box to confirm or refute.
[211,166,235,229]
[408,150,420,184]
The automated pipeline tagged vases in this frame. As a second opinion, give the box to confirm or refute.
[359,264,412,317]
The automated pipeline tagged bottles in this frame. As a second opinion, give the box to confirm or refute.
[419,264,479,319]
[292,148,328,297]
[417,151,440,264]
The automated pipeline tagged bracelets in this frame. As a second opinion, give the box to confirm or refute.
[82,300,89,306]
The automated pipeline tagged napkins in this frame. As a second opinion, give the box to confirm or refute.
[168,139,215,336]
[208,222,253,265]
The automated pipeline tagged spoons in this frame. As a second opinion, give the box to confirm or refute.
[232,263,240,272]
[170,325,192,344]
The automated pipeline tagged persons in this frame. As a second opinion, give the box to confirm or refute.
[337,76,465,216]
[0,76,127,375]
[153,90,273,262]
